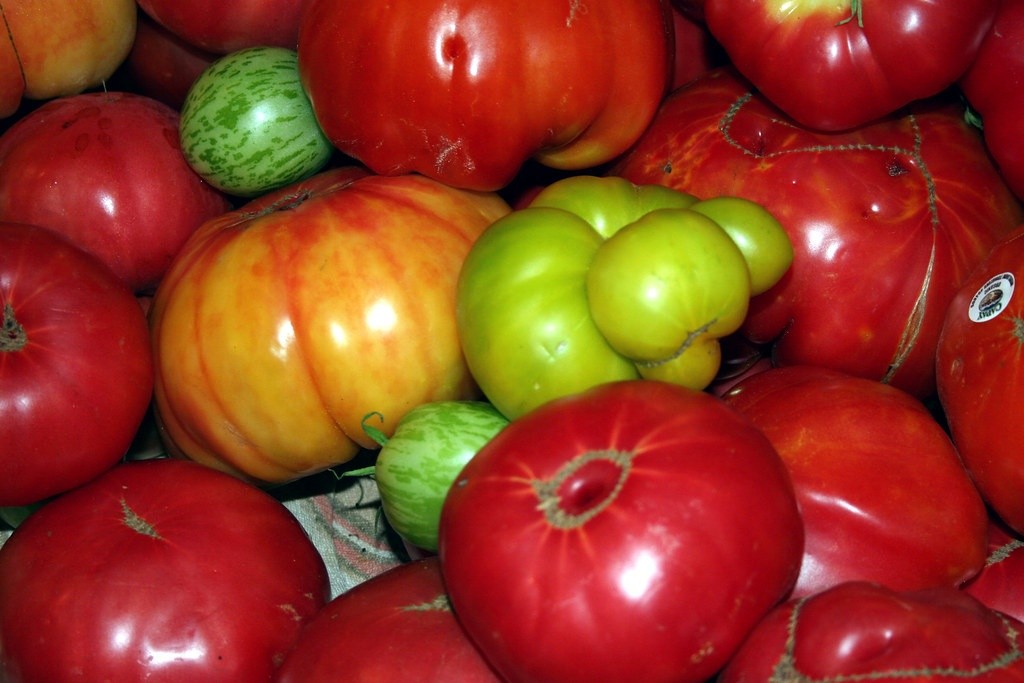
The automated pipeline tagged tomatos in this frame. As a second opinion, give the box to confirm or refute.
[0,0,1024,683]
